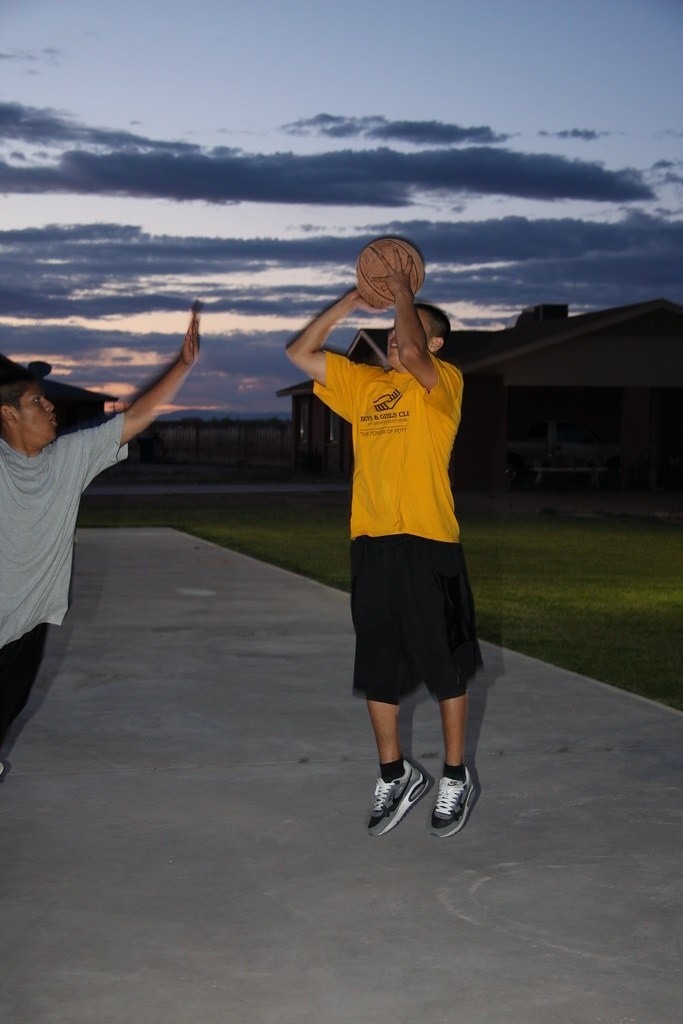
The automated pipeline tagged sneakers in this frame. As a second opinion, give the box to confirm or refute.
[429,767,475,838]
[368,759,428,836]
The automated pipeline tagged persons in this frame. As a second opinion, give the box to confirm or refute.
[285,247,486,837]
[0,311,199,776]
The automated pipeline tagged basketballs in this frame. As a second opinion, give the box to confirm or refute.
[355,238,427,309]
